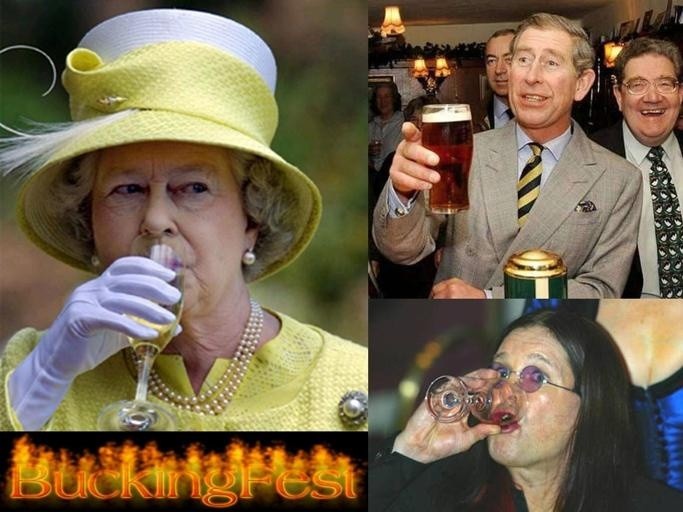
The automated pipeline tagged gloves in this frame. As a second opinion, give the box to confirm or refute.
[3,255,183,433]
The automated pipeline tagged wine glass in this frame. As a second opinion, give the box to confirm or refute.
[371,136,384,161]
[95,232,187,431]
[423,369,530,429]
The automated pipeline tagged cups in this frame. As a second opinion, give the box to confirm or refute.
[419,100,475,219]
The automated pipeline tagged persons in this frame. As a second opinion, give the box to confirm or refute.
[369,83,406,176]
[474,28,516,132]
[0,11,369,433]
[590,36,682,300]
[367,310,683,512]
[371,10,643,301]
[370,95,448,299]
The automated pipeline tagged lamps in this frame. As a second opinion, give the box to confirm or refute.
[412,56,451,97]
[602,42,624,68]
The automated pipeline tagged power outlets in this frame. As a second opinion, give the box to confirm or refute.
[366,5,406,67]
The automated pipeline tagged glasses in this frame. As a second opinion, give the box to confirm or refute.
[620,77,683,96]
[489,361,574,393]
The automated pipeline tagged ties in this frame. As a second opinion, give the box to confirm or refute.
[645,145,683,299]
[518,143,543,231]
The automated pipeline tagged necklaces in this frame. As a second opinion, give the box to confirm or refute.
[121,294,262,417]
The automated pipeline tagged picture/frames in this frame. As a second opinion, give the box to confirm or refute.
[477,72,490,106]
[368,74,396,120]
[618,0,673,39]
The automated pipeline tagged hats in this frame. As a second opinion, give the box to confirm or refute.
[1,8,322,281]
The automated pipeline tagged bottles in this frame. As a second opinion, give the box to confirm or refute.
[503,248,568,298]
[601,31,607,43]
